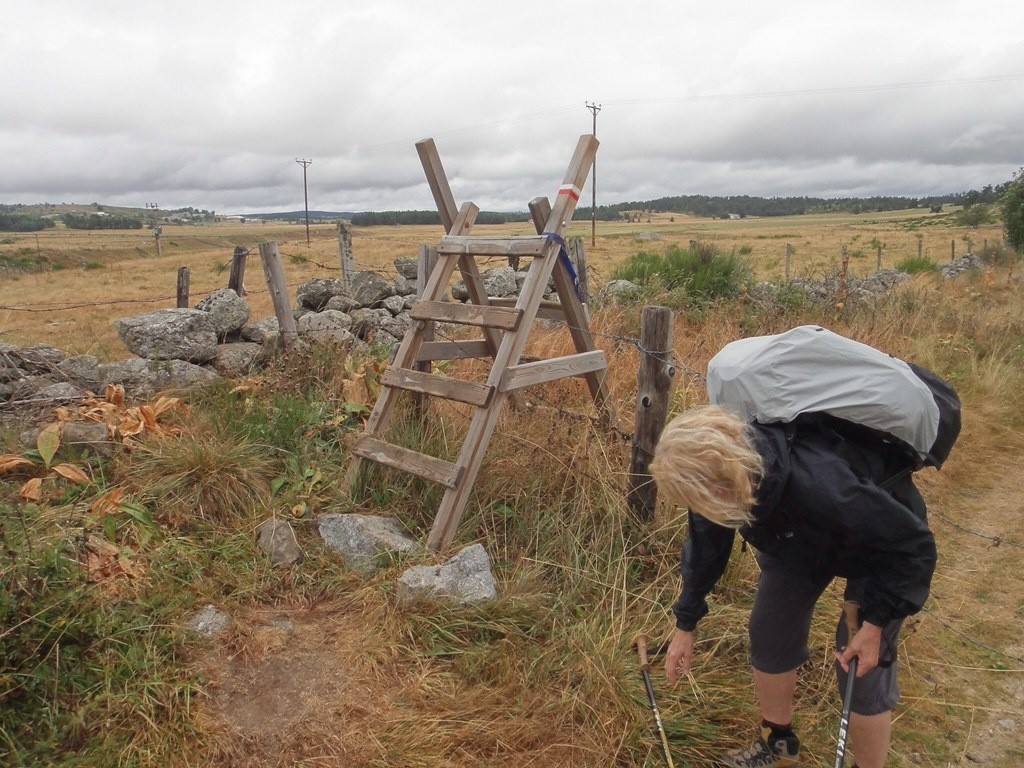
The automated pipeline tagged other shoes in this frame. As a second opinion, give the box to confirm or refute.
[703,725,800,768]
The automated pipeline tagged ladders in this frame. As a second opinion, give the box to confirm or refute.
[329,133,625,558]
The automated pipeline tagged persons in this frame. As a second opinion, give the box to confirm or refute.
[647,403,936,768]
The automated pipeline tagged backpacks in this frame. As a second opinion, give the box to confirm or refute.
[705,324,964,486]
